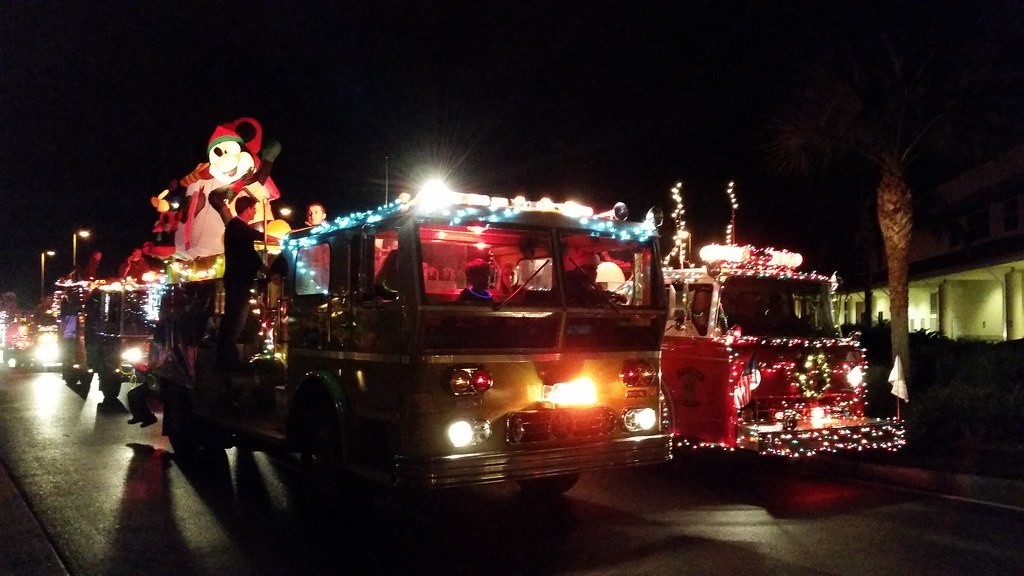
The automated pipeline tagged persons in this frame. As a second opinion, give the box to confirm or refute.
[561,253,612,307]
[374,249,400,305]
[127,323,181,428]
[457,259,497,305]
[307,203,326,226]
[498,262,527,304]
[214,195,284,375]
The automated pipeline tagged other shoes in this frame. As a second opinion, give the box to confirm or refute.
[140,413,157,427]
[128,417,143,424]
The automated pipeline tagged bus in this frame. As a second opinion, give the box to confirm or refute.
[661,264,906,459]
[133,187,675,511]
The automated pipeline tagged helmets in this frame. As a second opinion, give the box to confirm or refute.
[466,259,491,278]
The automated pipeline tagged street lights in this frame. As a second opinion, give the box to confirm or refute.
[41,250,56,299]
[73,230,91,267]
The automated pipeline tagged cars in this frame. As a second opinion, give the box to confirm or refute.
[0,277,162,397]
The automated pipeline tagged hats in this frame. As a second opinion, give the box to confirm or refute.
[575,251,600,266]
